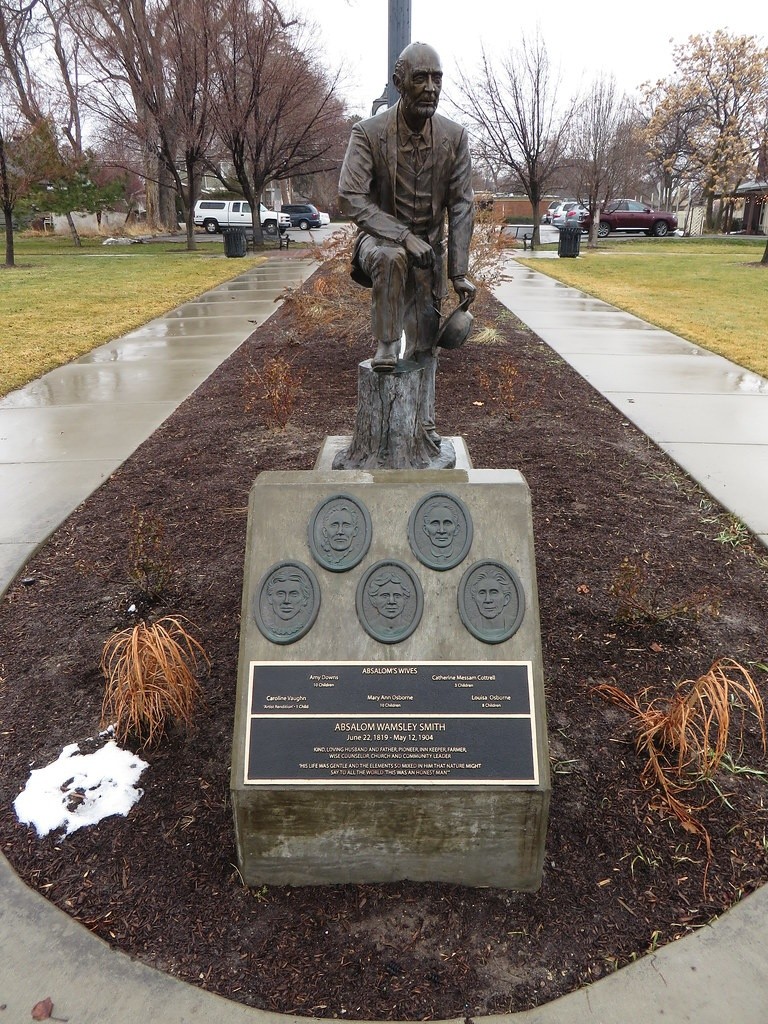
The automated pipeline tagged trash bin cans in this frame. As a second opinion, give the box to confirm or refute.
[219,226,247,257]
[558,226,584,257]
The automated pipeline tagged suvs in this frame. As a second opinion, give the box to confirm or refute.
[542,199,678,237]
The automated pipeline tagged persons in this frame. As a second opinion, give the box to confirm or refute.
[337,41,477,445]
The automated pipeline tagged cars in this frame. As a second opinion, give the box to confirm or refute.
[269,203,331,231]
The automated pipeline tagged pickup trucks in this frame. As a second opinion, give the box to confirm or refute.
[193,199,292,235]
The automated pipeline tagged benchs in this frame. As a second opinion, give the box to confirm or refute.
[245,227,290,252]
[498,226,538,250]
